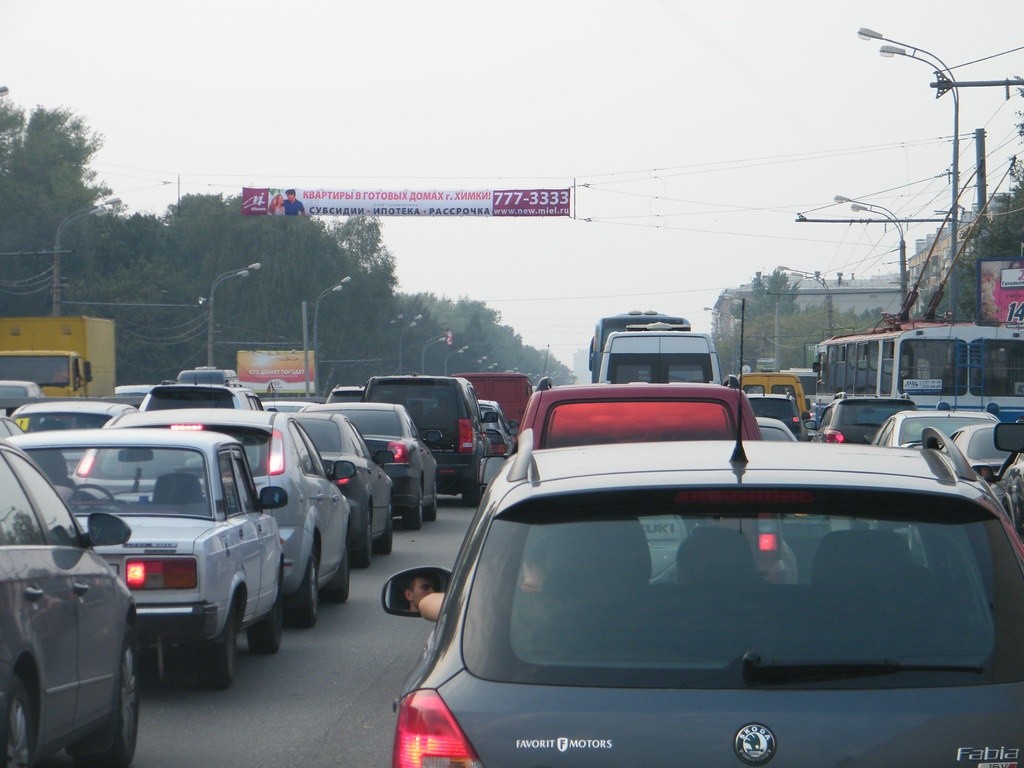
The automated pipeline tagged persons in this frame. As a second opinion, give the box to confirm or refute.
[283,189,305,215]
[403,575,437,612]
[268,194,285,215]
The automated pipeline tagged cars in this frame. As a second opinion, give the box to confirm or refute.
[756,418,833,570]
[0,444,141,768]
[783,368,818,408]
[379,426,1023,768]
[0,371,533,509]
[851,411,1001,531]
[5,430,289,691]
[943,425,1024,540]
[278,412,396,570]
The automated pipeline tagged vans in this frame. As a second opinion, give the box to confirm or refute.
[600,324,723,385]
[482,378,783,582]
[737,373,806,413]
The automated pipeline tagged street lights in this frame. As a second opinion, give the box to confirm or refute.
[777,266,835,339]
[398,315,488,378]
[314,276,351,393]
[208,262,262,367]
[52,198,122,319]
[855,27,959,321]
[833,195,909,321]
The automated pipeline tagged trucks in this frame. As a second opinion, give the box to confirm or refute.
[0,318,119,399]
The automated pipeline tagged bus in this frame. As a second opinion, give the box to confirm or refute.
[810,320,1024,429]
[587,308,690,383]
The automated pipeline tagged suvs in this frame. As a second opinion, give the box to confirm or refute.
[363,375,500,505]
[805,392,918,447]
[73,398,359,628]
[746,392,817,442]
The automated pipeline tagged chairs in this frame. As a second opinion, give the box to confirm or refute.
[811,528,920,599]
[547,511,654,592]
[153,471,206,506]
